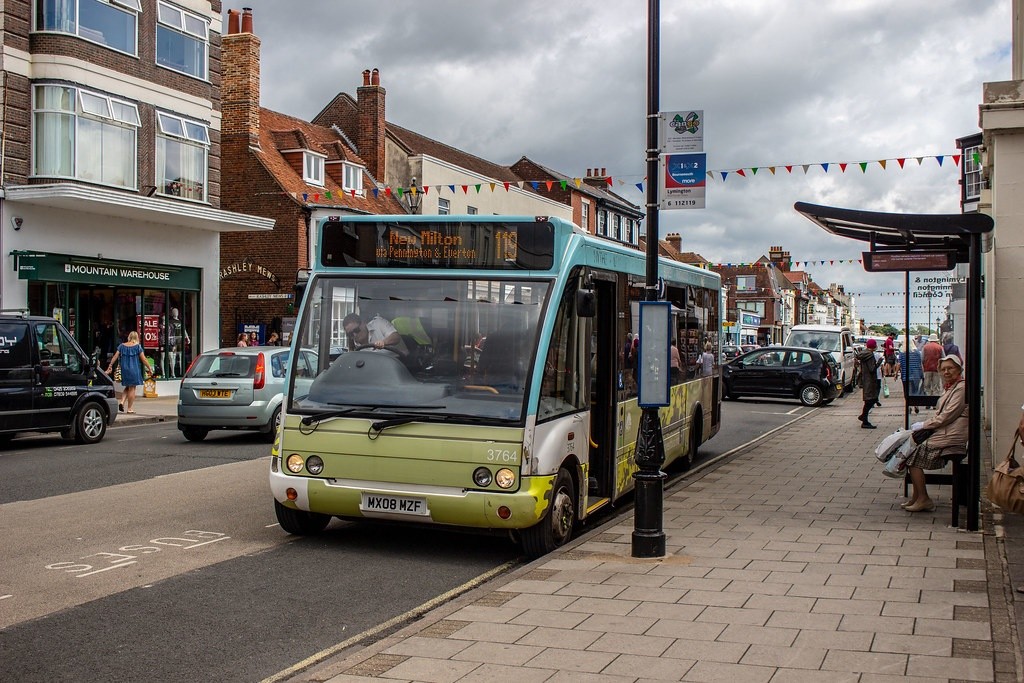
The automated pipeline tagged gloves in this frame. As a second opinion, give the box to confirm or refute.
[911,422,924,432]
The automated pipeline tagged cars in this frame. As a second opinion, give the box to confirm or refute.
[721,346,843,407]
[741,345,768,361]
[722,346,745,361]
[855,335,915,373]
[310,346,349,366]
[176,345,332,443]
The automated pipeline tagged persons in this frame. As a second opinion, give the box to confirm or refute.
[475,302,526,396]
[105,332,153,414]
[921,333,963,410]
[900,337,922,414]
[618,331,640,398]
[900,354,968,511]
[722,349,727,362]
[342,314,418,380]
[1017,405,1024,448]
[235,331,260,348]
[158,305,191,378]
[701,342,717,377]
[671,338,682,384]
[266,332,279,348]
[856,339,882,429]
[743,339,766,348]
[883,334,897,377]
[911,333,925,351]
[759,353,781,366]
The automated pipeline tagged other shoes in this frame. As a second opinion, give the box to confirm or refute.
[861,422,877,429]
[901,499,916,508]
[875,401,881,406]
[915,406,919,413]
[905,497,934,512]
[926,406,930,408]
[858,415,868,424]
[872,406,874,408]
[933,408,936,410]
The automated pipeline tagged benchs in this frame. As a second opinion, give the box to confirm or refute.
[947,454,968,528]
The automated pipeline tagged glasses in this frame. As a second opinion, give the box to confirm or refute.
[346,324,361,338]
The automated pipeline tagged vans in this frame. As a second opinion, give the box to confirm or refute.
[779,324,856,397]
[0,308,121,444]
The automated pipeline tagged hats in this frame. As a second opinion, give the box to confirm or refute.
[704,341,712,352]
[627,333,633,340]
[928,334,939,341]
[937,354,963,375]
[867,339,877,349]
[634,339,639,347]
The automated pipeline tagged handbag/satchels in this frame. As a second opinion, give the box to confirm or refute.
[987,428,1024,513]
[912,429,935,445]
[882,436,917,478]
[883,378,889,397]
[875,429,913,463]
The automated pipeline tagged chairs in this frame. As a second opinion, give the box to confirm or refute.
[391,316,432,374]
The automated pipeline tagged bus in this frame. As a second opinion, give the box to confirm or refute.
[268,213,723,556]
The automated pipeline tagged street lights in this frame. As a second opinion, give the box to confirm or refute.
[723,277,733,347]
[926,290,931,335]
[936,317,941,339]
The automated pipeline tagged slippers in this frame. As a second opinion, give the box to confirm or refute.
[119,403,124,412]
[127,410,134,413]
